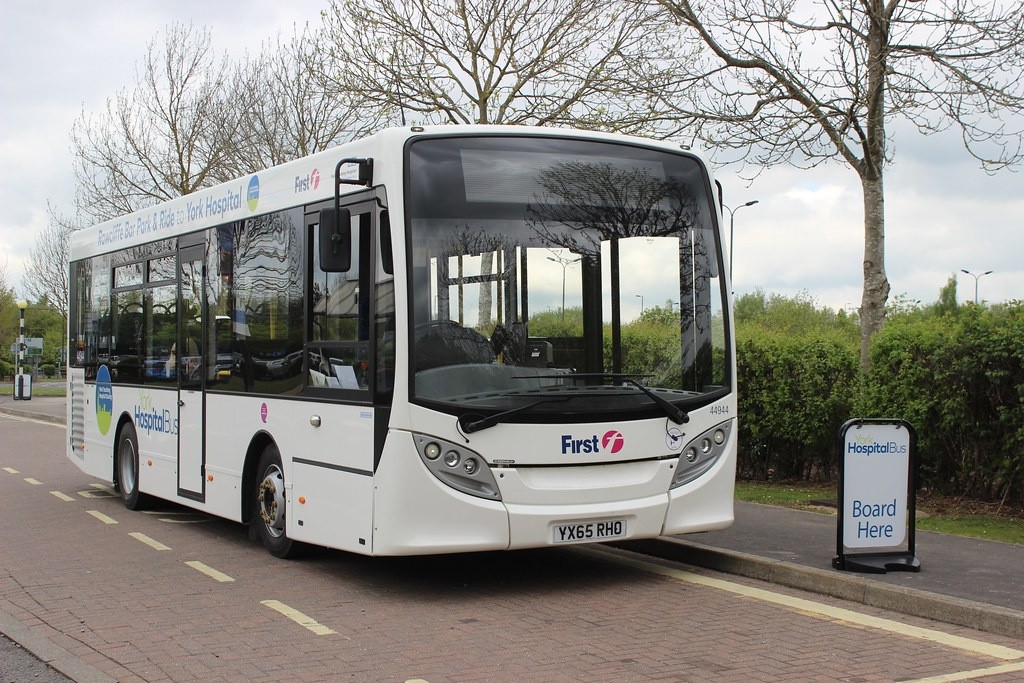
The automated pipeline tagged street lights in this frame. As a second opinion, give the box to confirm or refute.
[635,295,645,322]
[961,268,992,306]
[546,255,583,322]
[720,200,758,292]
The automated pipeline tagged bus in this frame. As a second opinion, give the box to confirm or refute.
[61,123,740,560]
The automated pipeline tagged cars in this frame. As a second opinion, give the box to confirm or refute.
[179,356,215,386]
[143,356,177,382]
[234,340,303,381]
[109,355,146,382]
[96,353,109,368]
